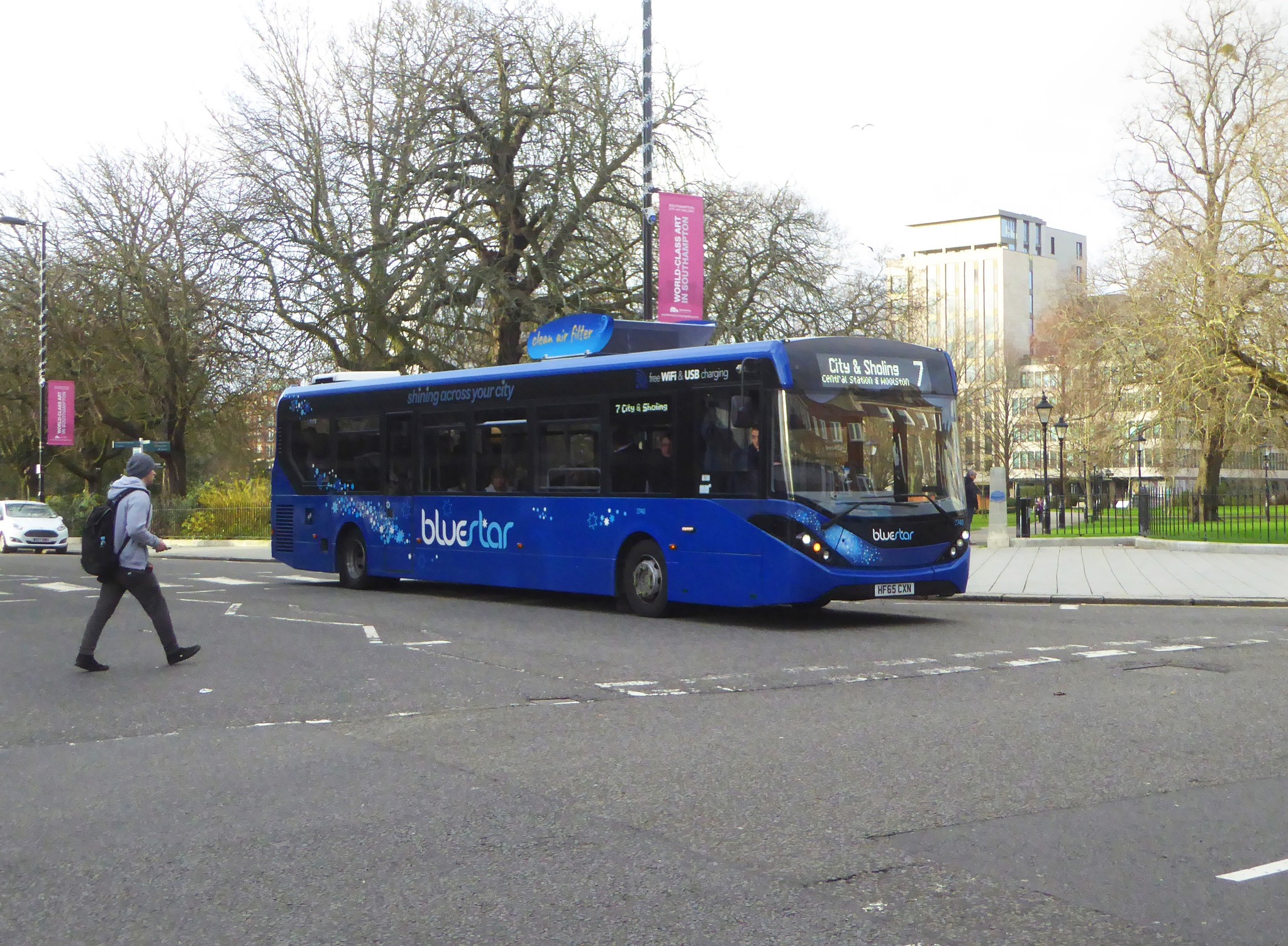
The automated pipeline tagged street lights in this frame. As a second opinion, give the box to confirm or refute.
[0,216,46,503]
[1079,444,1088,523]
[1035,389,1053,538]
[1131,429,1147,488]
[1092,452,1098,521]
[1262,455,1270,523]
[1053,416,1069,528]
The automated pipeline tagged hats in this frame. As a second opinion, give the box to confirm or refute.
[126,453,155,479]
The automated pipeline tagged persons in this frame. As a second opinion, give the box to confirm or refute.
[748,426,761,459]
[1036,498,1043,522]
[655,434,674,492]
[484,468,506,492]
[74,453,201,673]
[964,470,979,545]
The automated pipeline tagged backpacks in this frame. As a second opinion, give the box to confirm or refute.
[81,487,151,582]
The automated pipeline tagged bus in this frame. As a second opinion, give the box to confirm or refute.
[269,312,971,617]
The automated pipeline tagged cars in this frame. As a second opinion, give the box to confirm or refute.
[0,499,70,555]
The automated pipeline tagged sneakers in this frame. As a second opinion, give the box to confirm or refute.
[167,645,201,665]
[75,653,109,671]
